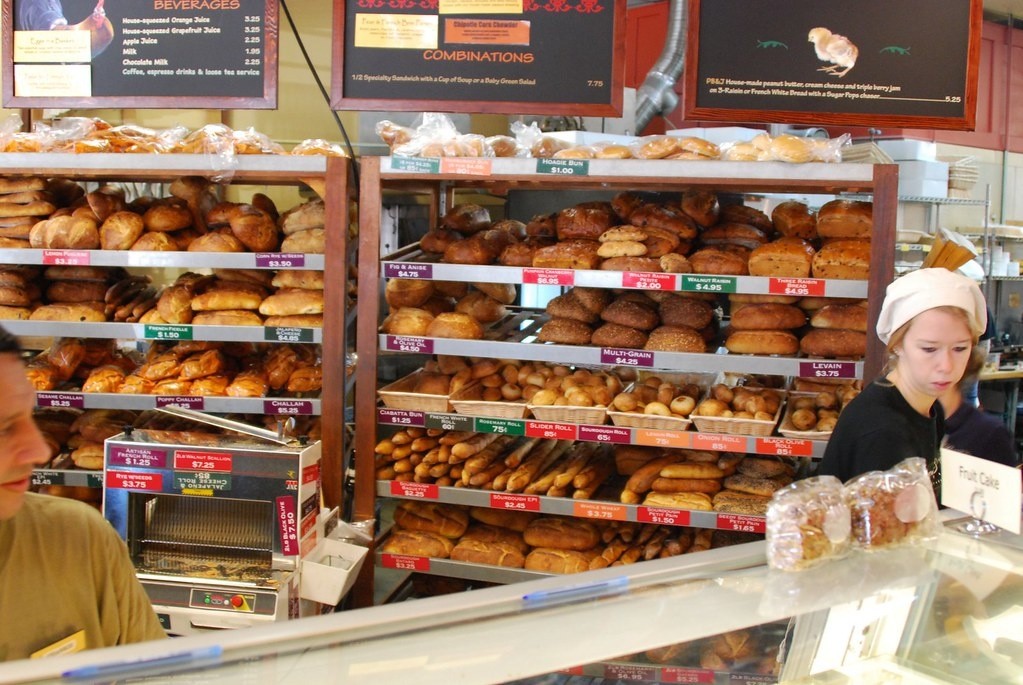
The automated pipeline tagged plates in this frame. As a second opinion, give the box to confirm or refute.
[897,229,925,243]
[937,154,981,200]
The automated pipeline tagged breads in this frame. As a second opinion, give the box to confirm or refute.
[380,277,871,361]
[375,428,811,513]
[0,107,349,156]
[0,263,321,325]
[24,337,358,397]
[372,119,843,163]
[0,168,329,255]
[759,485,852,569]
[393,354,866,435]
[845,473,927,547]
[418,188,872,281]
[604,626,789,677]
[34,410,355,525]
[382,500,756,577]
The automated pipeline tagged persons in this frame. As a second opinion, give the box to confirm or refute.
[0,323,171,665]
[818,267,1015,510]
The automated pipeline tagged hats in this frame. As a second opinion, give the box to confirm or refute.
[959,260,985,281]
[875,267,988,346]
